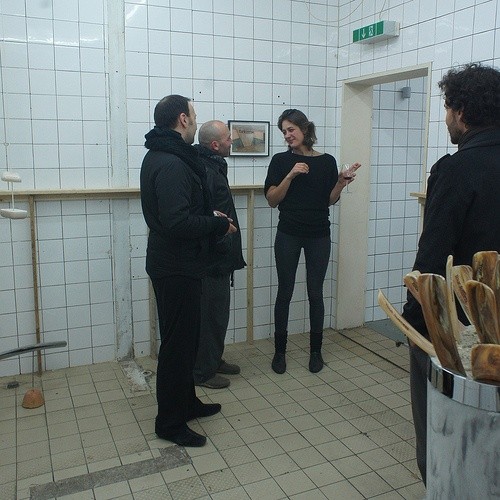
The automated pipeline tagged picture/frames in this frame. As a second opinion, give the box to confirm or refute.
[228,120,270,157]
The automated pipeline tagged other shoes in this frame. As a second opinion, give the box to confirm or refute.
[216,360,240,374]
[196,374,230,388]
[186,403,221,421]
[163,426,206,447]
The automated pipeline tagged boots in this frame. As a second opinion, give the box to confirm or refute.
[272,332,288,374]
[309,330,324,373]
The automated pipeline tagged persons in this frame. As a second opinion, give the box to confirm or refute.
[139,95,236,447]
[393,63,500,489]
[264,109,360,374]
[190,119,247,389]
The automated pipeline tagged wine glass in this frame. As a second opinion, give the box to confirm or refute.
[341,159,353,193]
